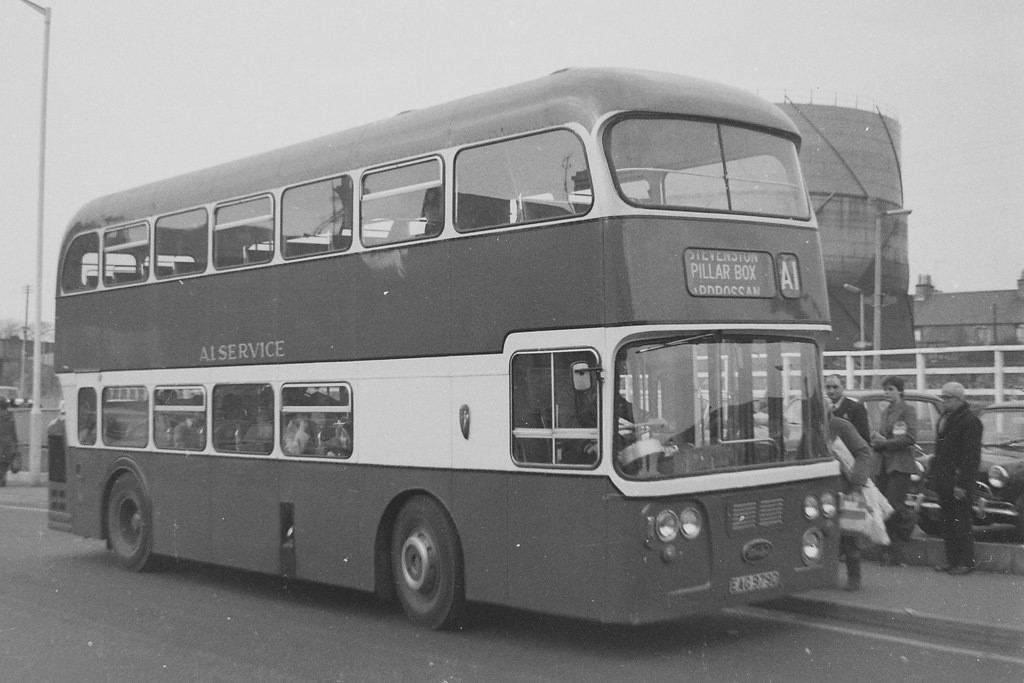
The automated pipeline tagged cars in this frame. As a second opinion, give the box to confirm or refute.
[783,389,1024,536]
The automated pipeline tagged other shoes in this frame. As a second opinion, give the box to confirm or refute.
[844,582,863,592]
[949,566,975,576]
[935,562,955,570]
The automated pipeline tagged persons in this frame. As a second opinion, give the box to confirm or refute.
[822,395,870,591]
[0,396,22,487]
[824,374,871,562]
[77,360,666,477]
[869,377,917,566]
[934,381,984,574]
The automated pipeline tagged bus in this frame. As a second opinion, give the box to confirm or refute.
[47,67,842,629]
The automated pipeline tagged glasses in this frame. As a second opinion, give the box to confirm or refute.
[937,395,957,401]
[825,385,840,390]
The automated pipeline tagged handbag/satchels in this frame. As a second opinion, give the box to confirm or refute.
[838,486,871,537]
[855,488,892,550]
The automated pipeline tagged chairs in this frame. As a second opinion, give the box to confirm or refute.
[142,266,173,277]
[86,276,113,287]
[173,261,205,273]
[245,250,274,261]
[217,257,241,267]
[113,270,142,285]
